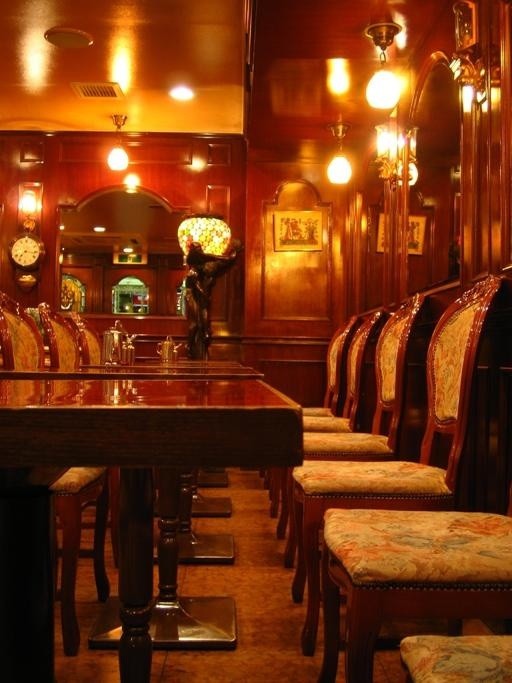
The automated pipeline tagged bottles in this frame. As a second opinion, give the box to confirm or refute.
[121,340,130,364]
[128,341,135,365]
[173,347,179,361]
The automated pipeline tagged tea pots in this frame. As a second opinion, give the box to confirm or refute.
[102,319,139,367]
[155,336,182,363]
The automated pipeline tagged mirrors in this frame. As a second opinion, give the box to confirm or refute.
[56,183,194,319]
[401,47,479,295]
[360,147,396,313]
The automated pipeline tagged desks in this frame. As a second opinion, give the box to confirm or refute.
[0,360,304,683]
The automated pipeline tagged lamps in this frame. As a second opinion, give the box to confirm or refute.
[18,189,41,231]
[325,119,356,186]
[409,123,420,187]
[372,122,397,180]
[107,111,130,172]
[362,20,405,113]
[176,212,247,363]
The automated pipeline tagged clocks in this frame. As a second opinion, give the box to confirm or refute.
[10,225,46,270]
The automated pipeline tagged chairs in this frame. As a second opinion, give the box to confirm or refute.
[289,268,511,658]
[315,505,512,680]
[274,291,428,572]
[269,306,390,520]
[257,314,361,490]
[0,292,111,662]
[394,632,512,680]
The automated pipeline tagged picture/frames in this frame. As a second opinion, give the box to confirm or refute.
[374,210,383,251]
[271,208,326,253]
[407,210,428,257]
